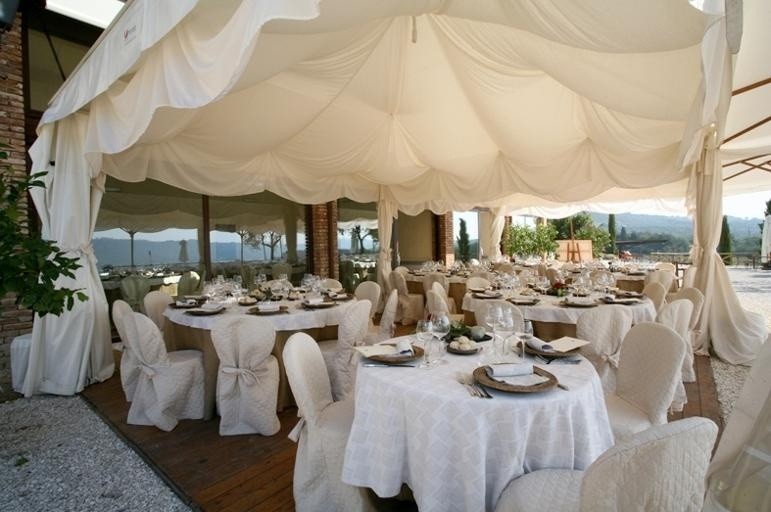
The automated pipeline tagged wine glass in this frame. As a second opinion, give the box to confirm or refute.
[203,274,328,308]
[416,303,533,369]
[425,253,660,299]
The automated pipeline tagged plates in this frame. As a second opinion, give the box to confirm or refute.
[168,290,354,316]
[469,284,644,307]
[366,334,581,393]
[408,270,469,277]
